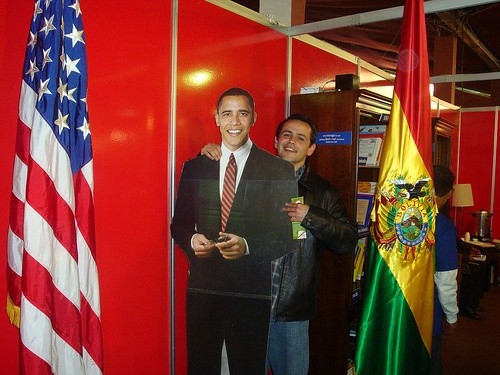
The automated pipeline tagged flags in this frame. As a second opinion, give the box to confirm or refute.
[6,0,104,375]
[353,0,435,375]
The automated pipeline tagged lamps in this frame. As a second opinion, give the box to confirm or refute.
[452,184,474,237]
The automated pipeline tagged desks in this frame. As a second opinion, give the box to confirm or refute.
[458,235,500,298]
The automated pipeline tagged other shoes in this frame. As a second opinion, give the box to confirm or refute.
[459,307,482,320]
[476,306,485,312]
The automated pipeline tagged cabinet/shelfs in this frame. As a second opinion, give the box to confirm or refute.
[292,93,392,375]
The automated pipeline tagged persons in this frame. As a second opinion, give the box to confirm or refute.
[432,166,459,355]
[200,113,358,375]
[170,88,298,375]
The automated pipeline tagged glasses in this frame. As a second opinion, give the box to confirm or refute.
[202,235,229,248]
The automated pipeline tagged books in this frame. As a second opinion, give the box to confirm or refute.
[354,126,388,283]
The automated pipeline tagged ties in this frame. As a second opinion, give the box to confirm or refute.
[220,153,237,232]
